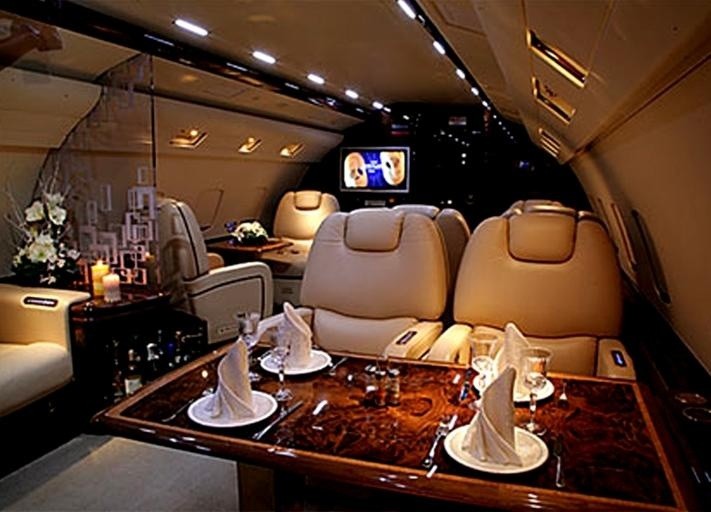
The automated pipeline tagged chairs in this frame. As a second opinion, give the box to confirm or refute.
[158,197,273,346]
[255,206,452,365]
[392,202,472,276]
[427,205,636,381]
[0,284,91,417]
[261,190,340,306]
[501,198,566,218]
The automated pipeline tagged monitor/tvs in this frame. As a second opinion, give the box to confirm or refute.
[339,144,414,197]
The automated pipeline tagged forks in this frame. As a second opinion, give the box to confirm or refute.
[556,375,571,410]
[420,412,459,469]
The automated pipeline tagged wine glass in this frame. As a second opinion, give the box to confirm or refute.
[518,346,554,437]
[234,310,261,383]
[467,333,500,416]
[269,330,296,403]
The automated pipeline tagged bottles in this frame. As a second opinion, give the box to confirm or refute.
[363,357,405,408]
[109,323,196,402]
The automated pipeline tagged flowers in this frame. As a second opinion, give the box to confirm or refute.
[2,161,82,287]
[223,216,269,245]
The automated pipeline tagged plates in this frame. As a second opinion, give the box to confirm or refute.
[444,422,551,475]
[258,344,335,377]
[186,388,279,428]
[472,366,557,403]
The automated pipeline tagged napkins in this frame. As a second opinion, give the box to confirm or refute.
[490,323,539,397]
[279,301,315,370]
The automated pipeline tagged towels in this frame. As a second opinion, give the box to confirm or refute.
[204,334,257,420]
[460,365,523,466]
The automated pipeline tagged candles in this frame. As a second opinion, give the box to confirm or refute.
[102,273,122,303]
[90,259,109,296]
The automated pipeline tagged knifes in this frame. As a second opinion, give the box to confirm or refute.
[550,432,567,489]
[459,366,474,406]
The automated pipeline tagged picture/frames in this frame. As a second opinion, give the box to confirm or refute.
[76,166,157,286]
